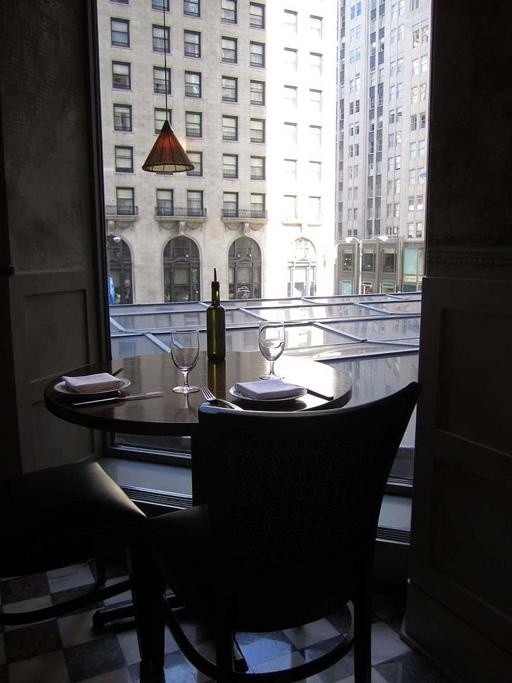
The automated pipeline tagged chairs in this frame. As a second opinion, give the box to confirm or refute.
[0,460,146,625]
[126,382,428,683]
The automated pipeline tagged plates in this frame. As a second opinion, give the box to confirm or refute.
[54,378,132,394]
[229,381,307,402]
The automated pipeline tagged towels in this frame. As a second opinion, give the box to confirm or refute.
[234,380,304,400]
[62,372,123,393]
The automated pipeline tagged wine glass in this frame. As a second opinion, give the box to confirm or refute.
[175,394,198,423]
[170,329,201,394]
[258,320,286,382]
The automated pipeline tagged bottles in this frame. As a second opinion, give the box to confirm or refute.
[207,360,226,405]
[206,267,227,360]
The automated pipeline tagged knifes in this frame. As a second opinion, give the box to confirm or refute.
[73,391,167,407]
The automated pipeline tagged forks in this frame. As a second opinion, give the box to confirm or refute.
[201,385,245,412]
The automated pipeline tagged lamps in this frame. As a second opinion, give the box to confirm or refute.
[143,0,194,173]
